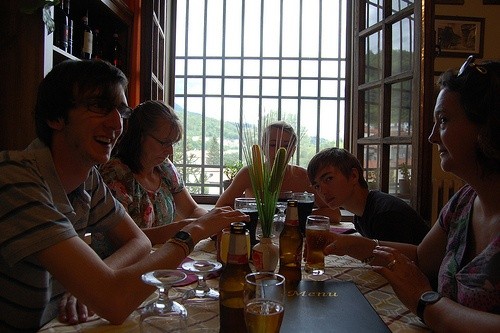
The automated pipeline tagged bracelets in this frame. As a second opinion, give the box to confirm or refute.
[366,239,379,263]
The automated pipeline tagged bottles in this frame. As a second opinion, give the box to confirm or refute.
[277,200,304,285]
[55,0,120,68]
[219,222,256,333]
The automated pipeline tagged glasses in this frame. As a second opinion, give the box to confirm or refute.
[74,100,133,119]
[456,55,500,86]
[142,130,177,149]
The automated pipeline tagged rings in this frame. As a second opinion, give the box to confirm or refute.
[391,260,397,264]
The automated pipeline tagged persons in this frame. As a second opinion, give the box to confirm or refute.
[1,61,251,332]
[214,122,341,225]
[91,101,207,258]
[307,147,429,247]
[304,55,500,333]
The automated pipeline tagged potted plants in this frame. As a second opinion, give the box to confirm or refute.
[233,111,308,278]
[223,160,243,191]
[398,163,411,195]
[367,171,378,191]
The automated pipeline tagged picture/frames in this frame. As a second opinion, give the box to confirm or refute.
[435,15,485,59]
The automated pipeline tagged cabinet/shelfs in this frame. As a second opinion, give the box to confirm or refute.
[0,0,166,152]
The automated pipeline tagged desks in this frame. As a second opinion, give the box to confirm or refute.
[38,222,431,333]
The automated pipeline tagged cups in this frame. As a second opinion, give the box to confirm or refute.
[243,271,286,333]
[234,191,318,236]
[305,215,332,276]
[220,229,252,265]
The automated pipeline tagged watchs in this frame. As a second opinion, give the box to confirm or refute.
[167,230,194,258]
[416,291,446,322]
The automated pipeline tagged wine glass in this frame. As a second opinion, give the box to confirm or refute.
[140,269,188,332]
[182,259,222,325]
